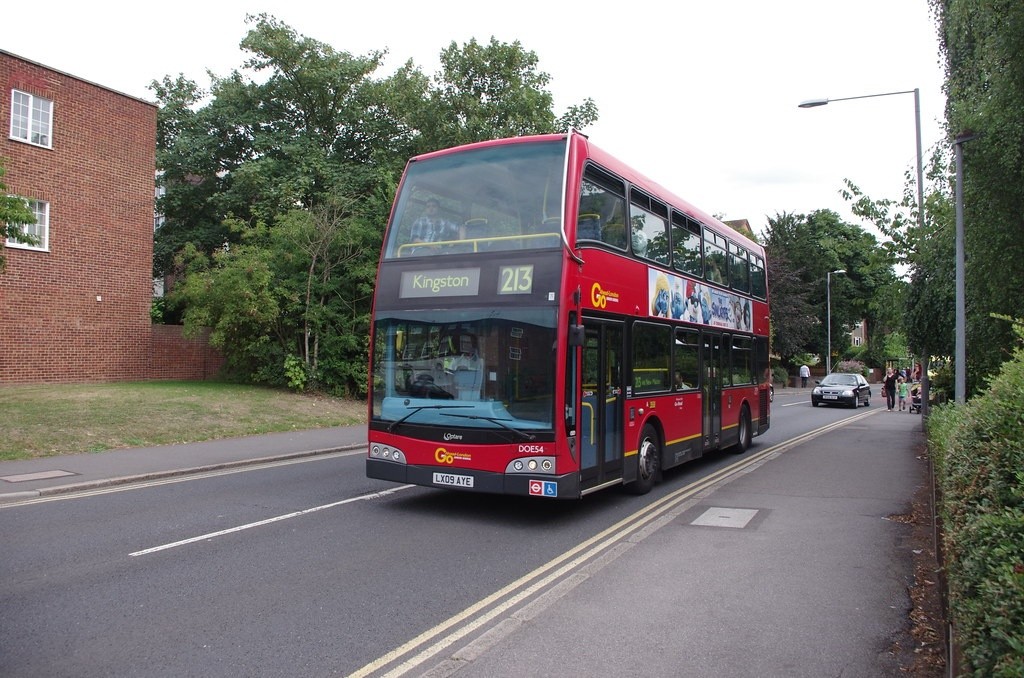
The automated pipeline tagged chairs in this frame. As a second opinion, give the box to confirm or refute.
[483,362,753,400]
[461,213,763,298]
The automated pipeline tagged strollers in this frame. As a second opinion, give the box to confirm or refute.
[908,382,922,414]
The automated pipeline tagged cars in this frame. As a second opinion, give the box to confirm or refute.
[811,373,871,409]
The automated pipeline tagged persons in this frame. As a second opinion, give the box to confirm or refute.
[883,363,922,411]
[409,198,466,254]
[799,364,810,388]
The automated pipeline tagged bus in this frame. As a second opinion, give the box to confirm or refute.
[367,132,772,510]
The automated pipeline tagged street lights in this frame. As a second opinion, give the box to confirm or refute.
[827,269,847,376]
[796,88,932,431]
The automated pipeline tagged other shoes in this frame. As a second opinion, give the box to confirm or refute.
[887,409,891,412]
[892,408,894,411]
[899,409,901,411]
[903,407,905,410]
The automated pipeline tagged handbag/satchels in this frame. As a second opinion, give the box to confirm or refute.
[881,385,887,397]
